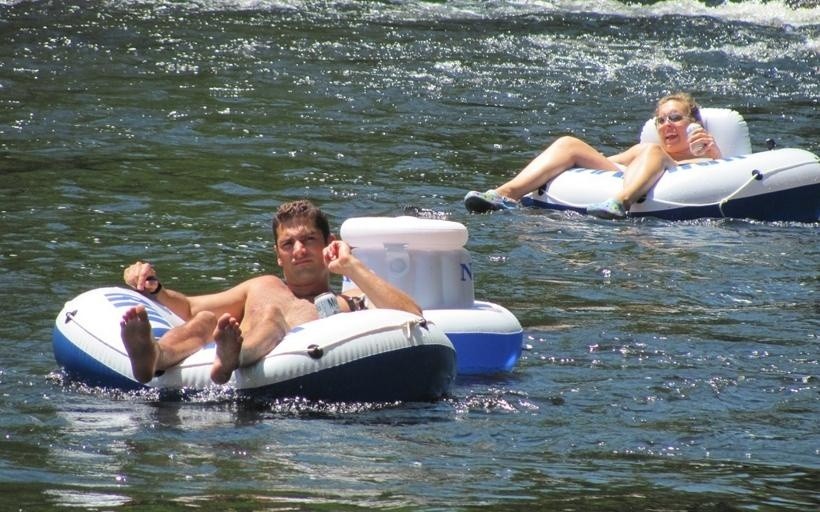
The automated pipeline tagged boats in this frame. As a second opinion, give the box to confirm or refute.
[44,209,528,413]
[519,94,818,230]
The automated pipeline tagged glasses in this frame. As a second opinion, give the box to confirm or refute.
[655,114,683,124]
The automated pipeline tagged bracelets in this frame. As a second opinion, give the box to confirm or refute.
[150,282,162,294]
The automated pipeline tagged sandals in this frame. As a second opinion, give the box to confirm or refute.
[586,201,626,220]
[463,189,519,213]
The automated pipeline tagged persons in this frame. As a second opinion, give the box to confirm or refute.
[464,93,723,219]
[120,201,423,384]
